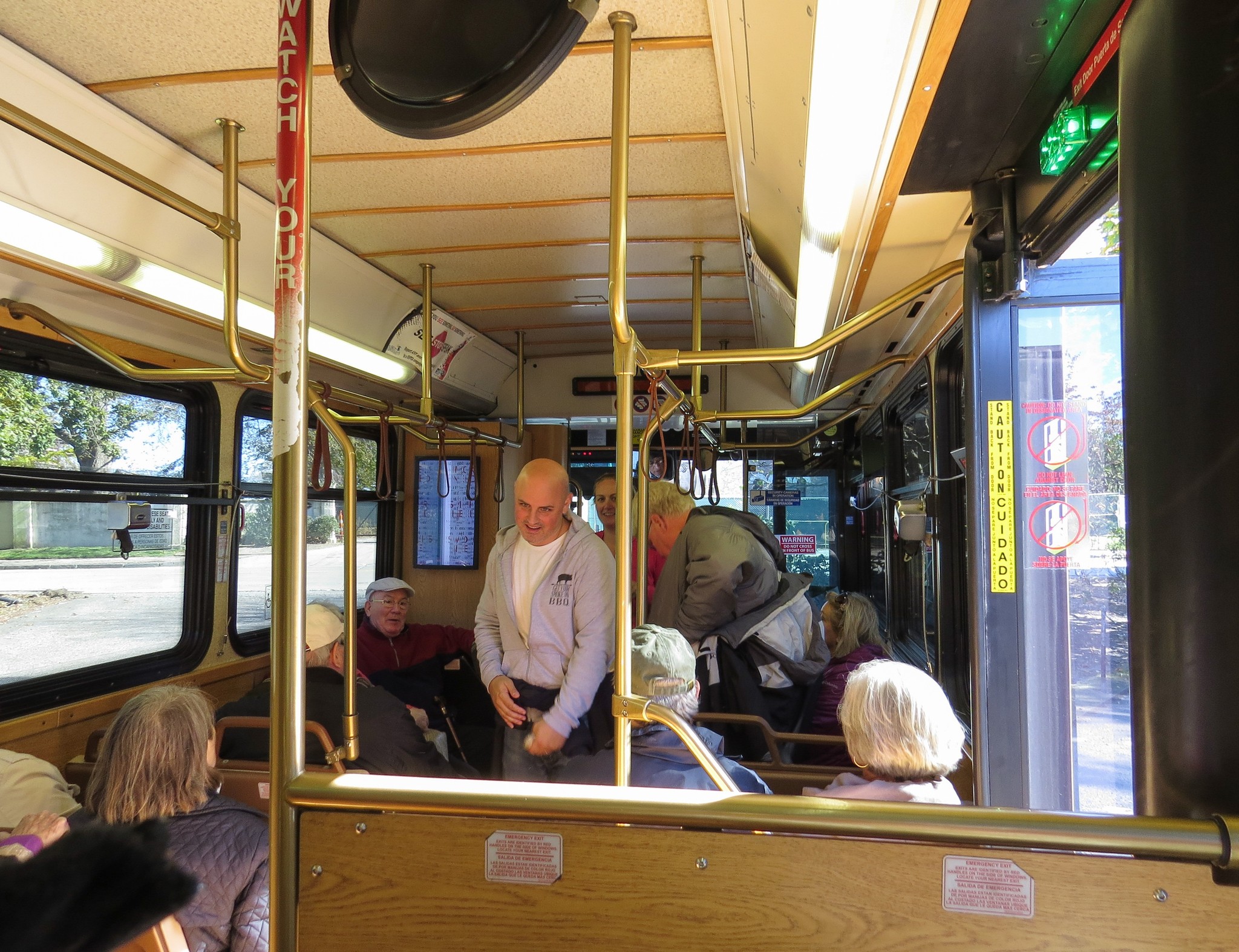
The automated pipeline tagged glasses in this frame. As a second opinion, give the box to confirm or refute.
[647,520,657,551]
[835,591,849,614]
[649,459,664,468]
[369,598,412,610]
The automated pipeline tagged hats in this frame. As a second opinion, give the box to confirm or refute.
[306,604,344,652]
[365,577,415,601]
[607,623,696,696]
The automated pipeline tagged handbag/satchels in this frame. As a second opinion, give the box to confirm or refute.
[548,672,613,758]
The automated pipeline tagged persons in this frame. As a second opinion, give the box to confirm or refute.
[212,602,460,782]
[473,458,614,783]
[795,589,894,769]
[649,454,664,478]
[553,622,775,795]
[590,471,668,629]
[794,502,836,544]
[631,479,831,764]
[81,683,272,952]
[307,596,428,733]
[0,811,71,883]
[796,658,968,806]
[355,577,482,780]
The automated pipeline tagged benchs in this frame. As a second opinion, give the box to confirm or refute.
[63,713,346,813]
[692,710,868,794]
